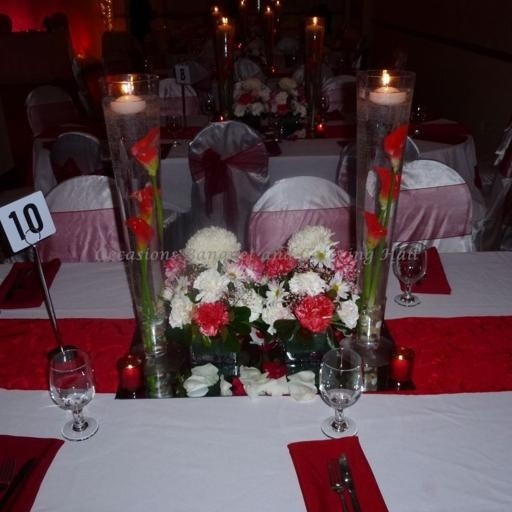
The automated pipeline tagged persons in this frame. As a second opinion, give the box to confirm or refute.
[311,32,362,82]
[0,13,93,176]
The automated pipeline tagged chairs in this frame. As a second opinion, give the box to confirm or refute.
[26,24,512,258]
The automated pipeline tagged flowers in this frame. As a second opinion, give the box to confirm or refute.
[127,125,167,354]
[357,122,409,337]
[160,223,362,396]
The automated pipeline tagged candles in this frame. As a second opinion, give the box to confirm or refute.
[263,0,281,16]
[305,16,324,32]
[211,5,233,33]
[390,355,409,382]
[123,364,141,389]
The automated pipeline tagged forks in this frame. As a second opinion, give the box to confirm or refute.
[326,460,348,512]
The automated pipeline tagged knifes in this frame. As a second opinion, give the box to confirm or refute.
[338,452,362,512]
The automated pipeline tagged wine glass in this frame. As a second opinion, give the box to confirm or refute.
[317,348,364,440]
[47,348,99,443]
[392,242,428,307]
